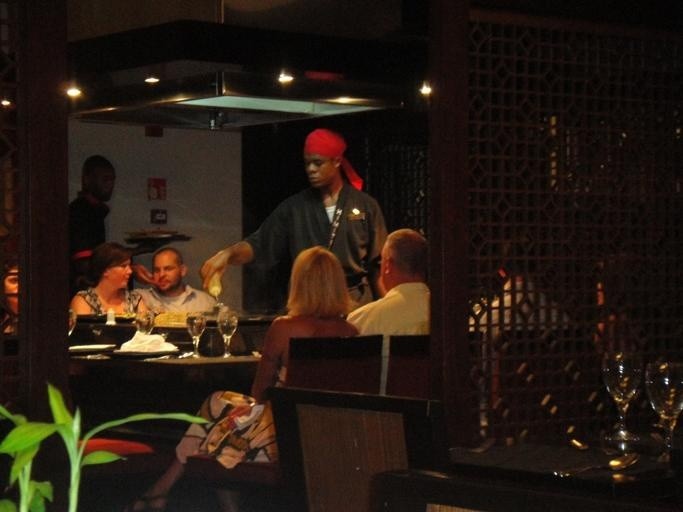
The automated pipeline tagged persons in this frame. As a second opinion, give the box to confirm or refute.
[345,228,431,396]
[464,231,574,400]
[66,241,151,335]
[117,245,358,512]
[132,247,225,316]
[199,126,387,309]
[67,154,164,300]
[0,256,20,333]
[589,261,634,361]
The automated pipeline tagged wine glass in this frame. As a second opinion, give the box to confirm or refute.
[215,310,238,359]
[184,311,207,359]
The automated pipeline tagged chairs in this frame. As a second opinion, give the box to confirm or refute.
[23,329,448,512]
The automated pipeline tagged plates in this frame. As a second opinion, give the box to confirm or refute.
[69,344,117,352]
[113,349,181,357]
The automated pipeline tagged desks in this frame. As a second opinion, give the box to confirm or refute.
[410,424,682,512]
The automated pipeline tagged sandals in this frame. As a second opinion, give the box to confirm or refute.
[121,494,173,512]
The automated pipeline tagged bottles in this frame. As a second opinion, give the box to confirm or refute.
[210,272,222,297]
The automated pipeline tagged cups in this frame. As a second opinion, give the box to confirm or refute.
[134,309,155,336]
